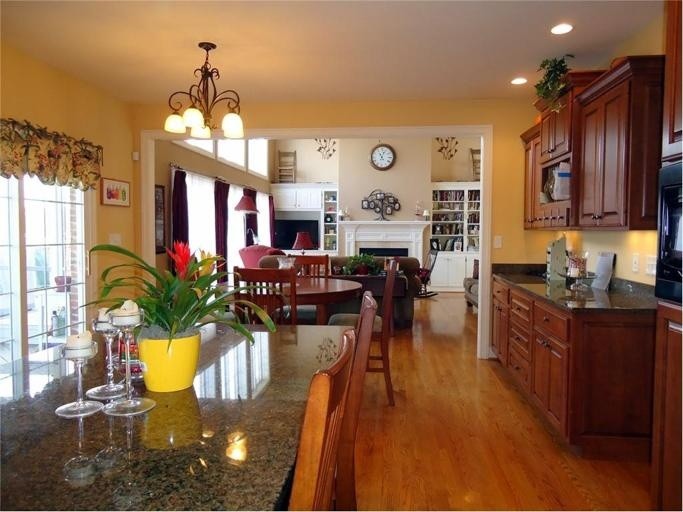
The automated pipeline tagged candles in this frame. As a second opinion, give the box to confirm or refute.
[107,298,141,326]
[91,304,120,333]
[60,329,94,349]
[569,266,580,277]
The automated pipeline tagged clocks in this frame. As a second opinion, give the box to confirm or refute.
[369,143,396,172]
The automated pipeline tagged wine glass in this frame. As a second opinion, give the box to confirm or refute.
[60,341,96,415]
[568,250,589,290]
[109,310,142,411]
[91,319,122,397]
[65,417,144,509]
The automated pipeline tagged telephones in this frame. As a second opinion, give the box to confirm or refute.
[433,224,443,234]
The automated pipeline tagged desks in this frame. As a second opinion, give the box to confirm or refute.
[0,317,360,510]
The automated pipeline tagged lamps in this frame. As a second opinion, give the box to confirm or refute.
[158,40,246,143]
[290,228,315,276]
[421,209,430,221]
[337,209,346,220]
[233,194,261,246]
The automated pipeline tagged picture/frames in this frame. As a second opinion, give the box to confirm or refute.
[152,184,166,256]
[98,177,131,208]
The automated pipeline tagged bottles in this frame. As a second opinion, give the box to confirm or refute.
[116,327,141,384]
[544,167,570,200]
[276,257,296,269]
[324,193,335,249]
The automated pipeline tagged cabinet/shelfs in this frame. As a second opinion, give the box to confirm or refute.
[646,296,682,511]
[526,292,660,464]
[427,180,481,294]
[659,0,681,168]
[503,279,533,411]
[573,53,665,232]
[267,181,339,257]
[518,66,614,233]
[489,269,510,379]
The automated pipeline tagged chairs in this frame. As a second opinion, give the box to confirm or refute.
[416,247,443,300]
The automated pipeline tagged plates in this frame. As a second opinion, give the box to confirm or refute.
[85,385,133,399]
[54,400,102,418]
[101,398,156,417]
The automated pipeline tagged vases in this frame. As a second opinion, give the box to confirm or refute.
[131,321,207,394]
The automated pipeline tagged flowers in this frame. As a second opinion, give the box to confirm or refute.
[71,231,293,358]
